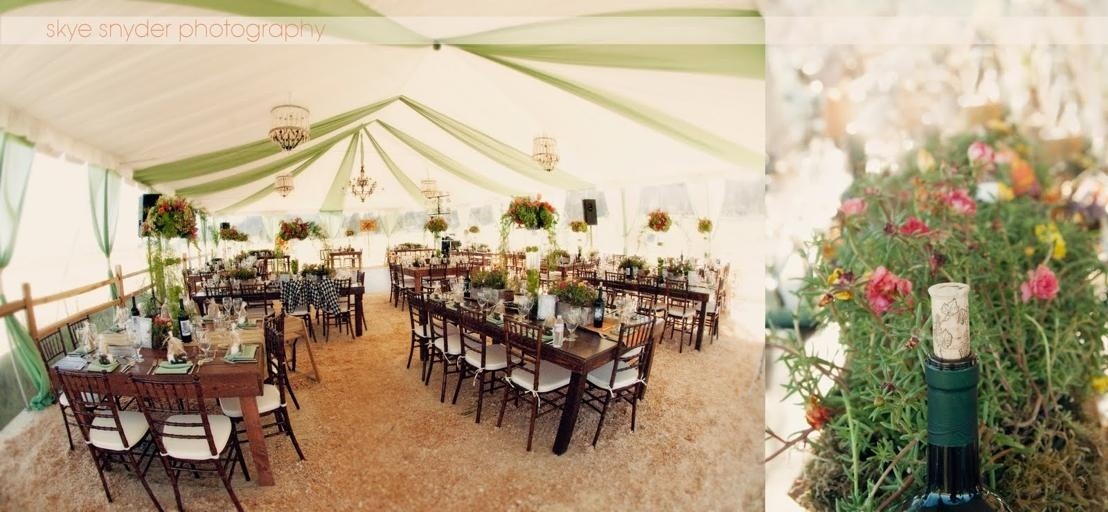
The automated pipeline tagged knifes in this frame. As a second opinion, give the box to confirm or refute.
[188,363,196,375]
[120,360,136,373]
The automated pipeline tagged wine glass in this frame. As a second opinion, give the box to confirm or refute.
[223,297,234,322]
[206,276,240,295]
[614,299,637,334]
[565,305,580,341]
[232,297,243,323]
[194,328,215,363]
[211,315,230,350]
[430,278,534,325]
[125,325,148,364]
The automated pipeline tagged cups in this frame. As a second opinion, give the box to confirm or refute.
[75,324,98,354]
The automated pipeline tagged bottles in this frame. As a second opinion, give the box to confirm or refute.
[593,286,606,327]
[129,296,140,331]
[906,362,1012,509]
[552,315,565,348]
[612,292,623,307]
[464,270,471,297]
[625,257,667,284]
[177,298,193,342]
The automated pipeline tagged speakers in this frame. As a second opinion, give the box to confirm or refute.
[220,223,229,229]
[583,200,598,225]
[138,194,162,238]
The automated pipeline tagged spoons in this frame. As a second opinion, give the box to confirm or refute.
[196,359,203,374]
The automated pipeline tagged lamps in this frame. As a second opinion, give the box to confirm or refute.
[419,161,437,205]
[533,125,561,175]
[270,163,294,200]
[261,77,315,154]
[345,130,375,201]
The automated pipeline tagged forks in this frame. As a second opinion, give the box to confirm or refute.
[145,359,157,376]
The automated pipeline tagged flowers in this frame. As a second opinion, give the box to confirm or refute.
[769,115,1108,481]
[129,189,716,254]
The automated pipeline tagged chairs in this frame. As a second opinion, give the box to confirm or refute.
[32,238,736,512]
